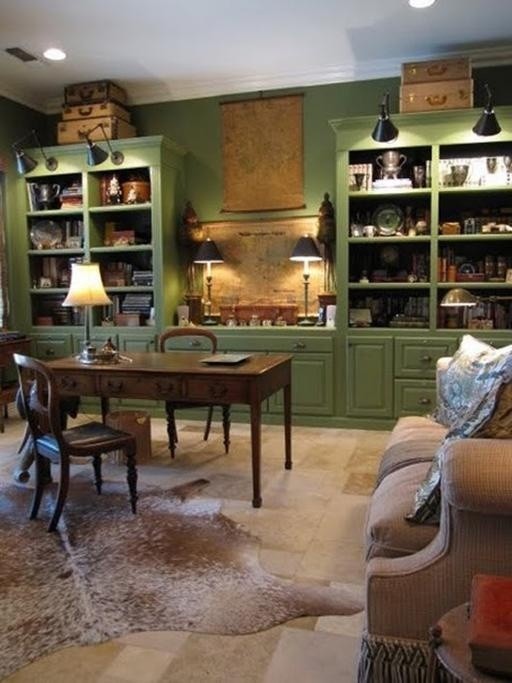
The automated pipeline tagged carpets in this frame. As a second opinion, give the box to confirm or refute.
[1,468,364,679]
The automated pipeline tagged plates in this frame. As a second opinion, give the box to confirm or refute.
[30,218,62,250]
[371,202,404,235]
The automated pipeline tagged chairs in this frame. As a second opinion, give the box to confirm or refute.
[156,327,233,459]
[10,352,139,532]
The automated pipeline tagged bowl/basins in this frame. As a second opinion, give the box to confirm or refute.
[456,274,486,283]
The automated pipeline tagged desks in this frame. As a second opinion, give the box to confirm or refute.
[46,351,294,507]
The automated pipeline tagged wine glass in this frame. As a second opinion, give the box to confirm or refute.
[353,173,365,191]
[414,165,425,187]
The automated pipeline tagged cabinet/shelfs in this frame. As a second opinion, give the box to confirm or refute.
[18,133,188,325]
[328,103,511,329]
[371,89,400,142]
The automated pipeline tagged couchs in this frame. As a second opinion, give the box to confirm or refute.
[358,353,511,682]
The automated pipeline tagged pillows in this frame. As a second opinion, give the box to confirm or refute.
[403,332,511,527]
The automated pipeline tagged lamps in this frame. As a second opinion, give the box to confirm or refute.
[289,234,321,324]
[12,127,58,175]
[471,81,501,135]
[192,239,225,323]
[63,261,114,364]
[81,122,124,167]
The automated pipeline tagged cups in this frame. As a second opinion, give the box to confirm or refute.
[452,165,468,186]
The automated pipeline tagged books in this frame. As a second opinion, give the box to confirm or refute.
[26,253,154,326]
[466,571,512,674]
